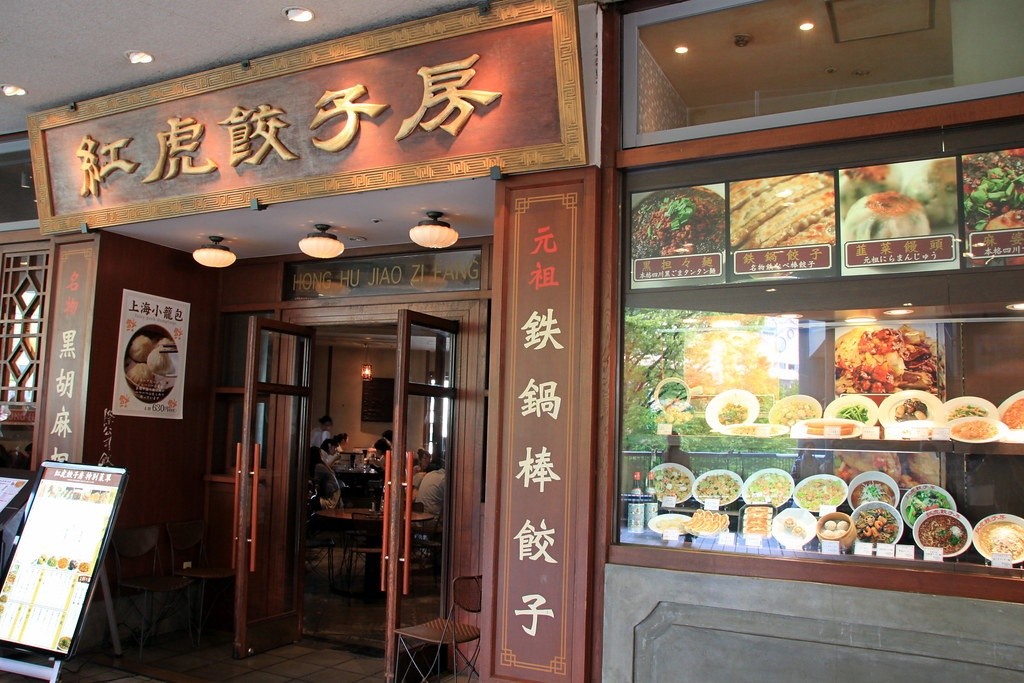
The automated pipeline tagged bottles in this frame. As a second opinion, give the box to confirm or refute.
[628,472,644,532]
[644,472,658,527]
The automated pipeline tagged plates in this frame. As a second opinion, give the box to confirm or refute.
[721,424,790,437]
[684,526,729,539]
[648,514,692,536]
[742,468,795,509]
[693,469,743,506]
[794,474,849,512]
[794,419,867,439]
[646,462,696,504]
[879,390,946,429]
[769,395,823,428]
[738,504,818,548]
[824,395,879,428]
[946,417,1008,443]
[944,396,999,422]
[997,390,1024,432]
[705,389,760,429]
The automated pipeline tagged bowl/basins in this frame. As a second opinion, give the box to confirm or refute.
[973,514,1024,563]
[848,471,900,512]
[900,484,957,529]
[913,508,973,557]
[851,502,904,545]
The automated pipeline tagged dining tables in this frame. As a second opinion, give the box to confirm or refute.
[332,466,382,507]
[314,509,433,595]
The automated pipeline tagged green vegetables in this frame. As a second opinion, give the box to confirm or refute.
[838,404,869,424]
[963,166,1024,232]
[938,530,959,546]
[908,491,950,525]
[867,486,881,497]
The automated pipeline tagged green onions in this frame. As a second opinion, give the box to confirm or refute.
[644,196,697,236]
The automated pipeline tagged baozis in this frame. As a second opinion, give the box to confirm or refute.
[127,334,174,386]
[822,520,849,537]
[837,157,957,240]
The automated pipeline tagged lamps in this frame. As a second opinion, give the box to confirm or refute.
[193,236,237,268]
[360,344,373,382]
[409,211,458,249]
[299,224,345,258]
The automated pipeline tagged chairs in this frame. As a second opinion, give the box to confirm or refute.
[307,507,443,599]
[100,526,195,662]
[389,575,482,683]
[154,520,235,651]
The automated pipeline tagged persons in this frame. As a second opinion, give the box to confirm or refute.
[333,433,348,451]
[320,439,341,468]
[310,447,340,509]
[310,416,332,448]
[791,442,819,480]
[412,447,443,541]
[664,432,691,469]
[374,430,392,472]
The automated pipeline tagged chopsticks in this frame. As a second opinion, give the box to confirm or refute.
[159,345,178,353]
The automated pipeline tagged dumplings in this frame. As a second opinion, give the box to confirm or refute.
[742,507,773,540]
[682,509,729,536]
[728,174,836,252]
